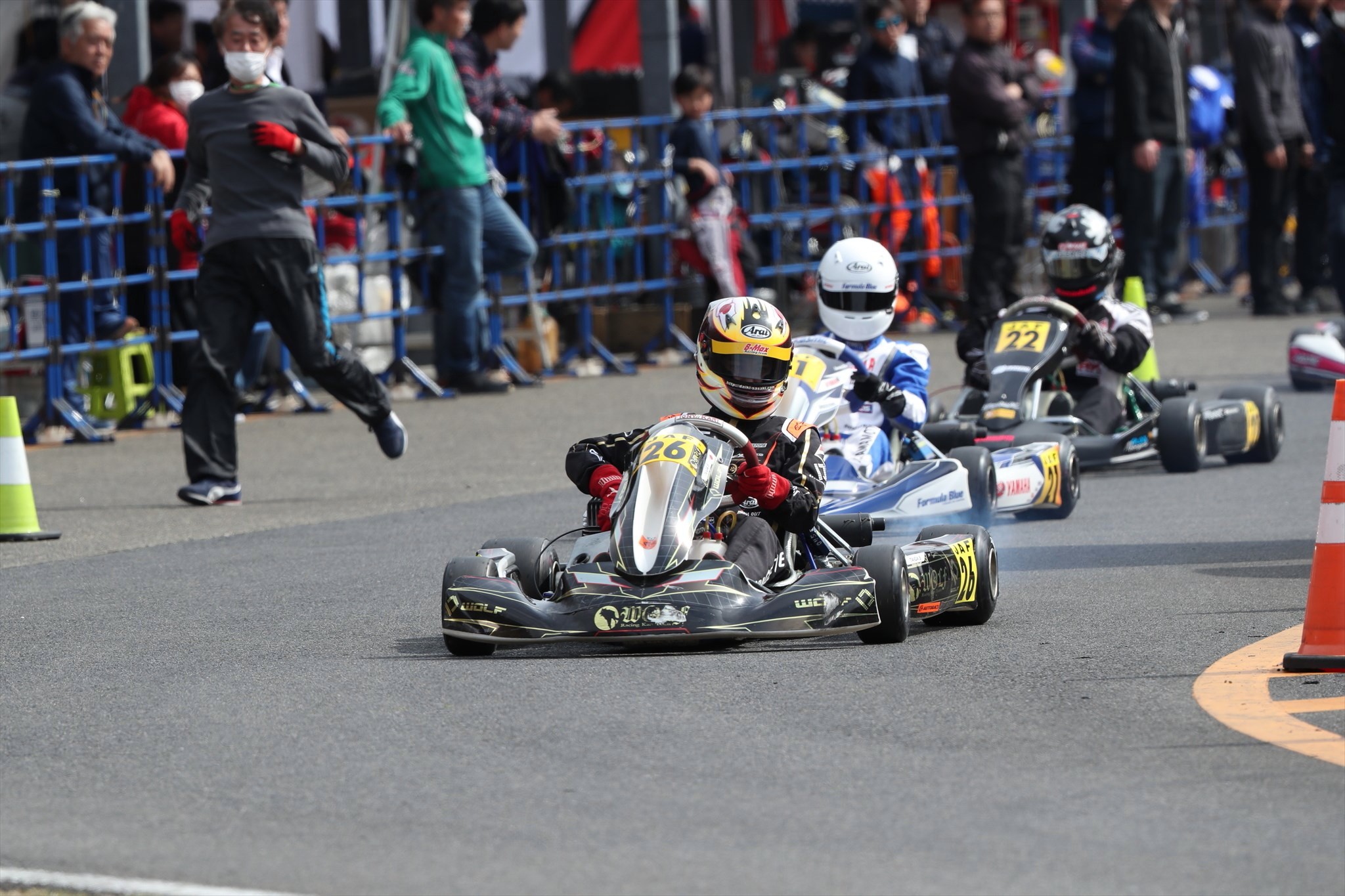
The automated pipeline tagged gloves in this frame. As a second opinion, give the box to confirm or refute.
[169,208,201,261]
[850,371,885,403]
[736,461,790,511]
[968,311,998,348]
[1075,319,1116,360]
[248,120,299,157]
[588,465,625,533]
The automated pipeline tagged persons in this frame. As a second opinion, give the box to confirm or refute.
[1068,0,1208,324]
[957,205,1153,437]
[169,0,409,507]
[664,62,747,297]
[16,0,350,428]
[765,0,963,336]
[814,238,930,481]
[1232,0,1345,316]
[565,296,827,586]
[375,0,574,392]
[946,0,1049,387]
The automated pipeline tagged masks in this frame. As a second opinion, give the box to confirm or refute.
[221,40,273,83]
[167,79,204,110]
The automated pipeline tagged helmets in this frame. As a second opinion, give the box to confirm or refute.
[1039,204,1125,303]
[814,237,900,342]
[696,296,794,421]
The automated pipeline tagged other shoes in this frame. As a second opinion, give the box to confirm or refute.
[438,364,508,394]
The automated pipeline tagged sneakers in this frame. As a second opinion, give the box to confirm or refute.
[368,410,408,459]
[178,477,243,507]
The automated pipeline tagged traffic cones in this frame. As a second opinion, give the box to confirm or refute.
[1281,376,1345,674]
[0,396,61,543]
